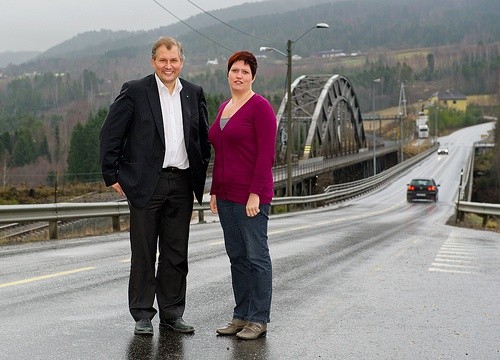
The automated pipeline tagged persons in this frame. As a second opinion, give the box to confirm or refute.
[207,51,278,340]
[98,35,212,336]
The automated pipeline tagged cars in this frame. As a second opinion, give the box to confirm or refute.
[438,146,449,154]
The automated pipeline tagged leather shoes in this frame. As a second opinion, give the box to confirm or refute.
[135,318,154,334]
[159,317,195,332]
[236,321,267,339]
[216,318,247,335]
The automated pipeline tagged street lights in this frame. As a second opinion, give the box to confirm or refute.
[372,77,381,175]
[259,22,330,211]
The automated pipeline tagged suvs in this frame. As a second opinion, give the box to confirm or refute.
[406,178,441,203]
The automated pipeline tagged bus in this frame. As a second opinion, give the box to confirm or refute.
[418,124,430,139]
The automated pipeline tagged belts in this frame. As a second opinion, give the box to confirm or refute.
[162,167,190,172]
